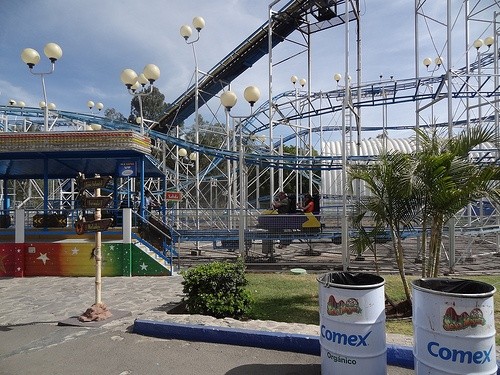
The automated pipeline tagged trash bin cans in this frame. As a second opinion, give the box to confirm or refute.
[316,271,389,375]
[410,278,497,375]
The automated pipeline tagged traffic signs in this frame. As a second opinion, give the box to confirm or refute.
[76,173,113,191]
[80,196,114,209]
[74,217,114,235]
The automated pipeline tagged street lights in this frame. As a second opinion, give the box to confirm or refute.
[178,147,197,239]
[423,57,444,131]
[472,37,495,224]
[8,99,26,210]
[120,63,161,135]
[21,43,63,131]
[220,86,261,262]
[179,16,206,250]
[290,75,307,209]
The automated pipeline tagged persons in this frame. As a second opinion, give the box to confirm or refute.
[120,190,162,213]
[273,191,315,215]
